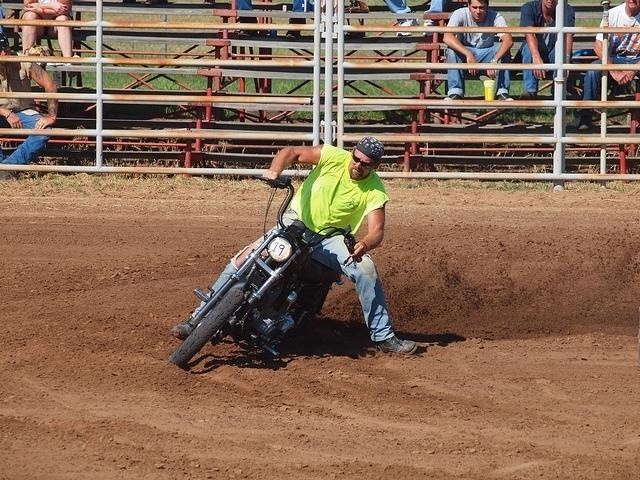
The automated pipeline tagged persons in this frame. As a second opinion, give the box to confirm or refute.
[573,1,640,132]
[16,0,83,79]
[517,1,583,100]
[382,1,448,38]
[442,1,517,102]
[232,0,311,40]
[169,136,421,358]
[0,31,60,184]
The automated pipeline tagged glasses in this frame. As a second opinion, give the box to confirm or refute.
[352,149,374,168]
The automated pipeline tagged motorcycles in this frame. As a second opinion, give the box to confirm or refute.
[166,172,362,370]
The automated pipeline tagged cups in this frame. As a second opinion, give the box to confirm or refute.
[484,80,497,101]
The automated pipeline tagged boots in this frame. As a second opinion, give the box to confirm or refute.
[565,110,594,133]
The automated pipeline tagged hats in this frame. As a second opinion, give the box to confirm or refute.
[356,137,385,163]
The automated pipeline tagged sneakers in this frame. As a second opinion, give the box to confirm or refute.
[0,169,19,181]
[444,94,463,101]
[238,25,435,39]
[494,93,514,101]
[520,92,538,100]
[377,335,418,356]
[171,310,198,341]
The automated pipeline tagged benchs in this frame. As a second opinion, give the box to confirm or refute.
[1,0,640,174]
